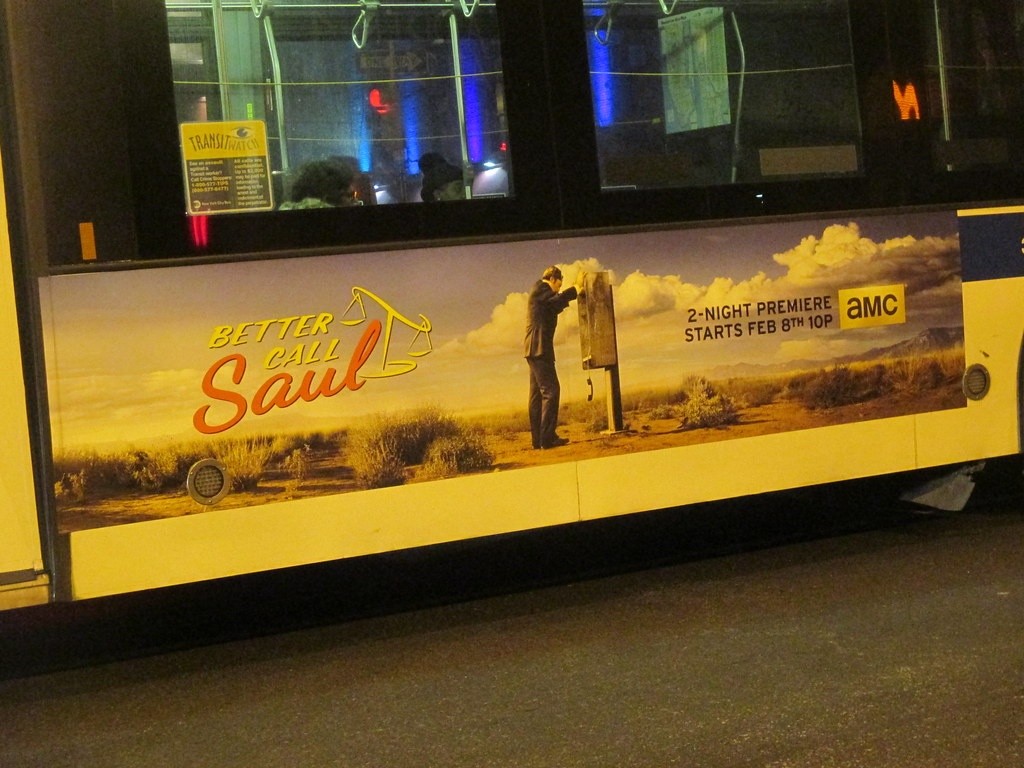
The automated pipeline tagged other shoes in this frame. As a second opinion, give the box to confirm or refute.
[543,438,569,449]
[532,445,541,449]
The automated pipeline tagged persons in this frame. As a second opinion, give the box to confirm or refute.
[277,136,475,210]
[525,265,587,449]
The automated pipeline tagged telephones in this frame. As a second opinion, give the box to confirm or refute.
[587,379,593,403]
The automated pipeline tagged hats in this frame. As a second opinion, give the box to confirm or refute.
[417,153,464,195]
